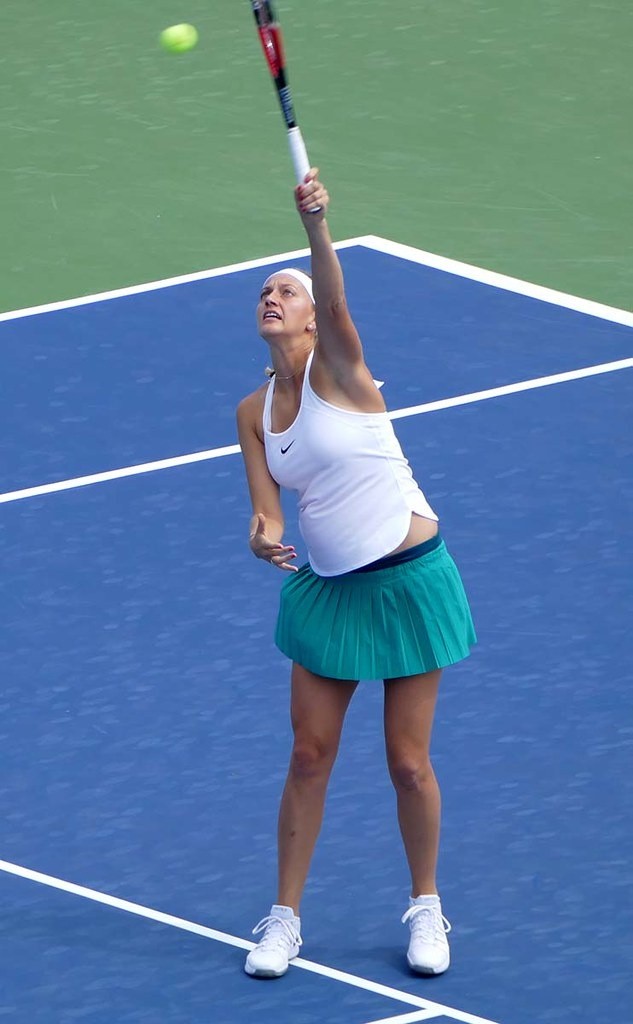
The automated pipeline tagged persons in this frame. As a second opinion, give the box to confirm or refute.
[237,166,481,976]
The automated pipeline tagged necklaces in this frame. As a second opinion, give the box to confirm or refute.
[265,365,306,380]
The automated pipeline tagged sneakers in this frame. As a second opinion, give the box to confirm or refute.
[401,894,451,975]
[244,905,303,978]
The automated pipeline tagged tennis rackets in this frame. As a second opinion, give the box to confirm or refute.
[250,0,323,217]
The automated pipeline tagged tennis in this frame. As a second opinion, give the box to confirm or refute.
[161,23,200,55]
